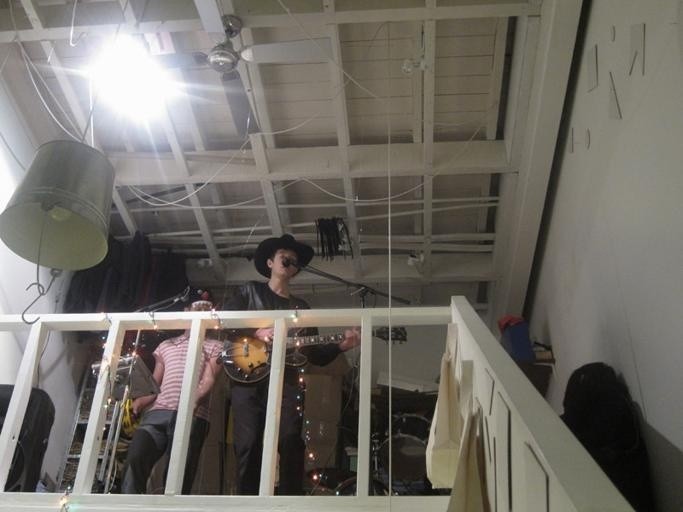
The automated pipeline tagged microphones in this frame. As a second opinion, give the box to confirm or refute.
[173,289,203,303]
[282,258,290,267]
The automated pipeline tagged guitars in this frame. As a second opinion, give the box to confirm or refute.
[222,328,406,385]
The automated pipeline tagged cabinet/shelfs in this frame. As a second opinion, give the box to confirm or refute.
[54,358,132,493]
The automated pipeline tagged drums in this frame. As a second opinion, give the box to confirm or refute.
[373,415,432,489]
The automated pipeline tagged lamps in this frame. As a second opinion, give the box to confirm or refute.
[0,0,151,271]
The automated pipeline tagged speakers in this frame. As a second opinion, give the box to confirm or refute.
[0,384,55,492]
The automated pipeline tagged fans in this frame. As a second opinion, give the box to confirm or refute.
[150,16,331,135]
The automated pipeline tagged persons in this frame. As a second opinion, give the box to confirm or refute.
[118,287,224,494]
[215,233,360,494]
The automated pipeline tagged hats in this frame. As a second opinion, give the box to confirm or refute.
[254,234,314,281]
